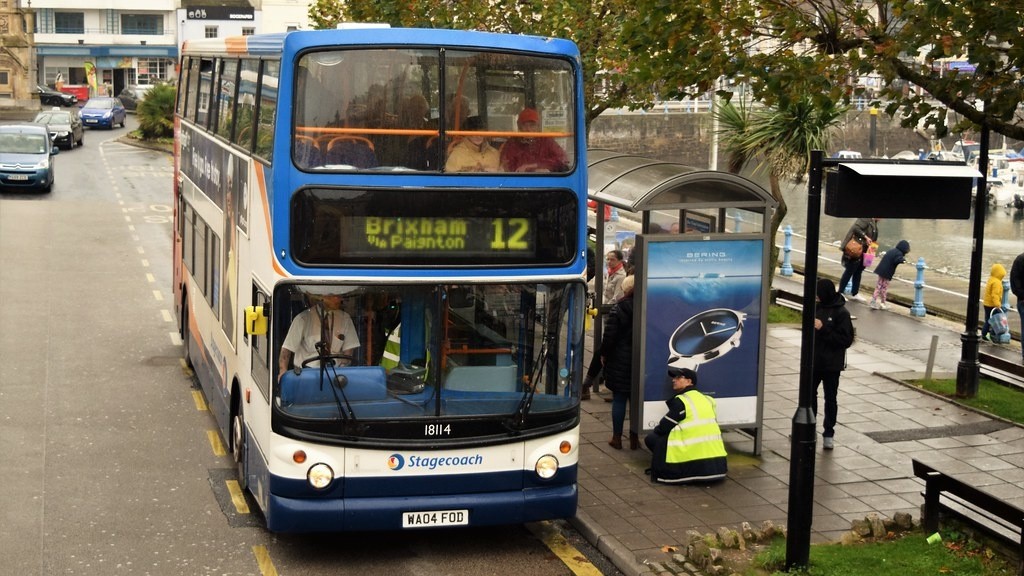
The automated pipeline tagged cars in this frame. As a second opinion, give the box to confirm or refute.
[0,125,59,193]
[119,84,154,110]
[78,96,126,130]
[34,107,84,149]
[37,85,78,107]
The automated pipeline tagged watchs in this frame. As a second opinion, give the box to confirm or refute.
[653,295,761,399]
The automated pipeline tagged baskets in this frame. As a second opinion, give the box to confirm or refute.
[987,306,1009,334]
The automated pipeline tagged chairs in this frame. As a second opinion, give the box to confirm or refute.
[239,105,506,170]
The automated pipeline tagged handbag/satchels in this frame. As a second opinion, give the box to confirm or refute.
[845,238,863,257]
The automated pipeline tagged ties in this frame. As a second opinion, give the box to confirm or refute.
[322,312,331,356]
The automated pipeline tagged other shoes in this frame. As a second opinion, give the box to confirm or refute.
[603,395,613,401]
[840,293,849,302]
[851,293,866,302]
[881,302,888,309]
[580,391,590,400]
[981,335,990,341]
[870,301,879,310]
[608,435,622,449]
[823,436,834,449]
[645,467,652,474]
[629,436,638,450]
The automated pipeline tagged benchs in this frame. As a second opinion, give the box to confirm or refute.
[771,287,858,322]
[977,350,1024,392]
[910,457,1024,564]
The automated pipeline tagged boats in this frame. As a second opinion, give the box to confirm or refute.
[830,141,1024,209]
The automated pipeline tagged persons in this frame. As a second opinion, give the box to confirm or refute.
[585,235,642,452]
[868,240,910,310]
[644,369,727,483]
[838,217,879,301]
[813,278,855,449]
[1009,251,1024,358]
[982,264,1007,344]
[277,82,567,393]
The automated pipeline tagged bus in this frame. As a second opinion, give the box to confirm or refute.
[173,16,587,533]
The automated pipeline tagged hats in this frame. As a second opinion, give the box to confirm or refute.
[462,116,486,131]
[667,368,697,385]
[517,108,539,124]
